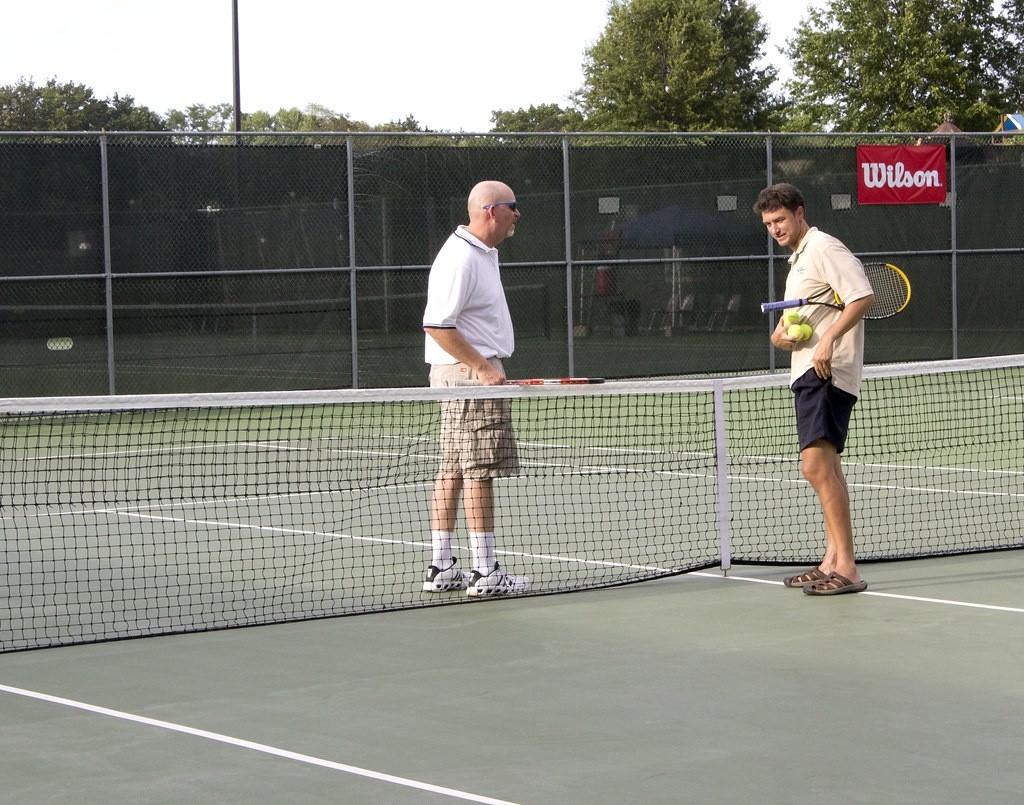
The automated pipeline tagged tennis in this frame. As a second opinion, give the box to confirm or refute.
[782,310,812,342]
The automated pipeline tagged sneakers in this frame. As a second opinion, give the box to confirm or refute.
[423,556,471,592]
[466,561,530,596]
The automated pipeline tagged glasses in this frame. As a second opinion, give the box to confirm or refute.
[483,202,517,212]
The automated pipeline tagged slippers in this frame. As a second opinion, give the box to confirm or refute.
[783,566,867,595]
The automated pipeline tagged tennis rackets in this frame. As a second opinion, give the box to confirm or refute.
[761,262,912,320]
[454,377,603,386]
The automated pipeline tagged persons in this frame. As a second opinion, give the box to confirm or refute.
[424,178,532,600]
[752,183,876,596]
